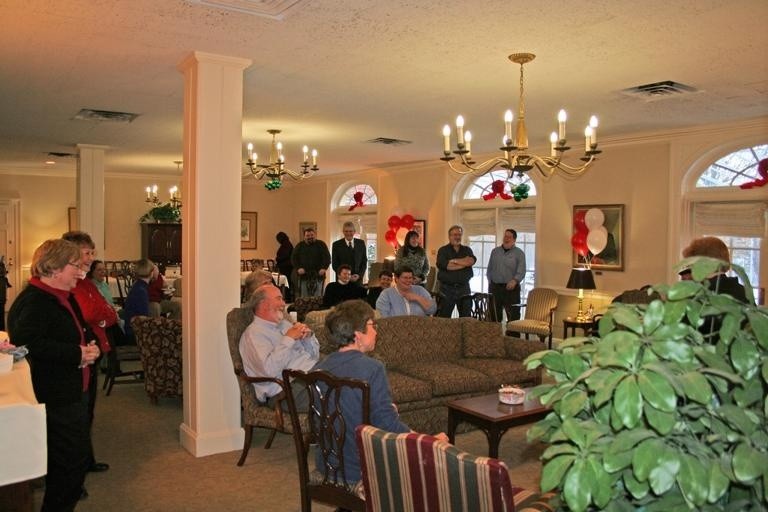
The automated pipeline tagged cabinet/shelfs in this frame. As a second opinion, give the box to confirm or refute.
[140,223,182,266]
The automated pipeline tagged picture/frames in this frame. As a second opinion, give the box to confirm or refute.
[241,260,274,271]
[411,220,425,249]
[573,204,624,271]
[241,211,257,249]
[299,221,317,244]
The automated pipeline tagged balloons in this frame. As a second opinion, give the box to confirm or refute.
[511,183,530,202]
[401,215,415,230]
[388,216,401,231]
[572,207,607,256]
[265,179,281,191]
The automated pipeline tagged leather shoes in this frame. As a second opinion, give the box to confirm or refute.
[91,463,109,471]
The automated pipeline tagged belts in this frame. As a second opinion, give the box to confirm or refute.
[445,283,468,288]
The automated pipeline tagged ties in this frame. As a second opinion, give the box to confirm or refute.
[348,242,352,248]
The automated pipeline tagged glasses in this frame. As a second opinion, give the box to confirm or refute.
[68,262,81,268]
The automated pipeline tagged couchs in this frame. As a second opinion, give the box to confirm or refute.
[311,316,546,435]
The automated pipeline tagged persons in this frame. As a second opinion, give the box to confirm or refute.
[0,230,171,512]
[678,269,692,281]
[308,299,451,485]
[239,285,322,413]
[244,221,527,339]
[683,237,757,346]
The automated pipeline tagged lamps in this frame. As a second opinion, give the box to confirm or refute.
[440,52,600,180]
[241,129,319,182]
[143,161,184,218]
[566,269,596,321]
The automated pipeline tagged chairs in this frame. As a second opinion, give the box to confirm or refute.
[112,261,174,319]
[102,316,181,406]
[226,308,319,466]
[432,290,499,320]
[506,288,558,349]
[297,268,326,298]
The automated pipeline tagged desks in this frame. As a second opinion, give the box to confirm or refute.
[564,315,593,340]
[240,271,289,303]
[1,331,48,511]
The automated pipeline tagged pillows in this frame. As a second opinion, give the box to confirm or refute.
[463,318,506,359]
